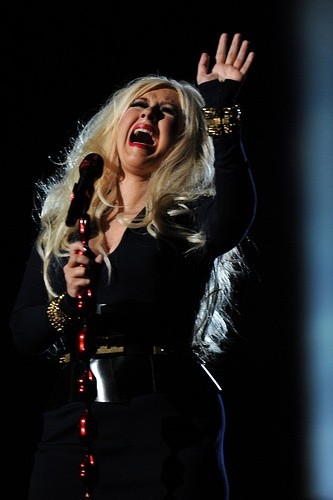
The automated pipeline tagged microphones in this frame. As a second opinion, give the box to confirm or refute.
[65,153,104,228]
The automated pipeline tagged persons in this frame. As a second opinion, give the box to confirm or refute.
[7,33,256,500]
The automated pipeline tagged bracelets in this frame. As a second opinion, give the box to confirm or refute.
[201,104,241,136]
[45,294,71,332]
